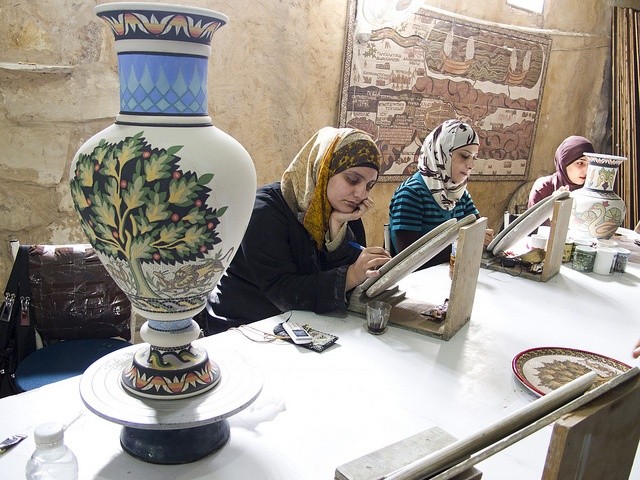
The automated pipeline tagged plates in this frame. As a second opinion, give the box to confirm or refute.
[512,346,634,398]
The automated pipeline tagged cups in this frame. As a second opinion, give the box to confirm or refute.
[530,234,547,252]
[365,300,392,335]
[562,230,632,276]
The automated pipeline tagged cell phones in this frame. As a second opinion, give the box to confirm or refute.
[282,322,313,344]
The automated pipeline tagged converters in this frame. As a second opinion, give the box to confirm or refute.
[500,257,515,267]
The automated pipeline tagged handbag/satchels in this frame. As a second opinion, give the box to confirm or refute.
[0,245,45,398]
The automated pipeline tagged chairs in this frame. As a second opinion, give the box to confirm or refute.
[8,239,141,393]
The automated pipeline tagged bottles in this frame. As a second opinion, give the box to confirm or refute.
[26,421,79,479]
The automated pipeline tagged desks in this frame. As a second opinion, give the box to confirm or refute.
[6,219,640,480]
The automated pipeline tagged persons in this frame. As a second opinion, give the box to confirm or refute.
[527,135,594,227]
[389,117,496,266]
[207,125,392,336]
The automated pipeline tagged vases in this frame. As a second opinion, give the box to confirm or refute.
[564,153,627,243]
[70,1,259,399]
